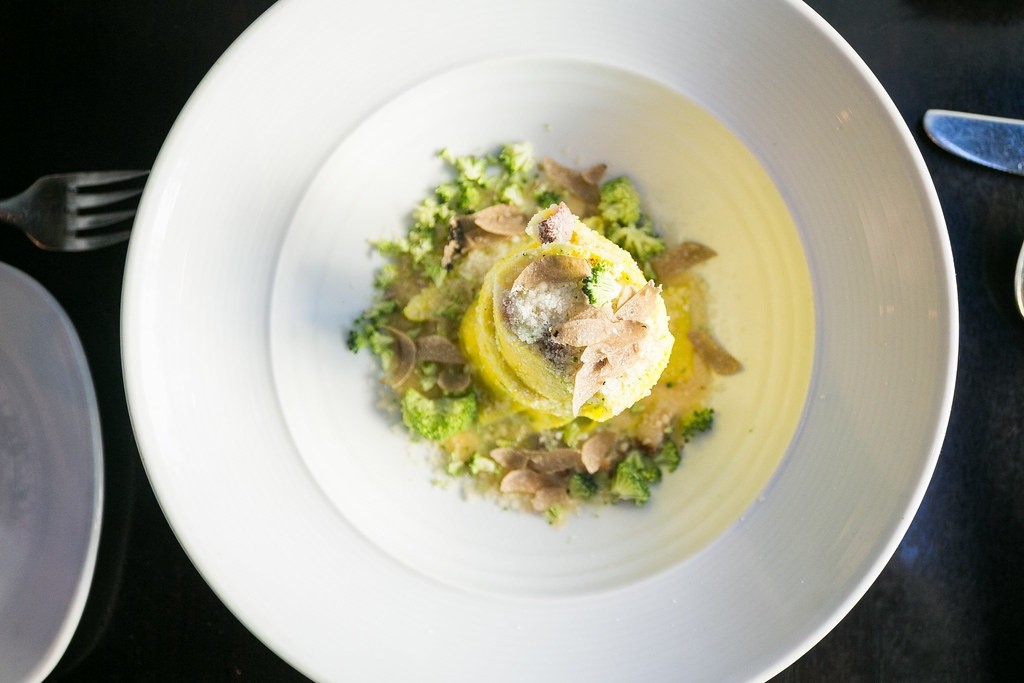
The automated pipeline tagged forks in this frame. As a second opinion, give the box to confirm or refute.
[0,168,163,253]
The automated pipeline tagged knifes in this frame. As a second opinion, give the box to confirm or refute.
[924,110,1024,173]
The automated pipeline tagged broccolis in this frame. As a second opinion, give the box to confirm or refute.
[343,145,716,526]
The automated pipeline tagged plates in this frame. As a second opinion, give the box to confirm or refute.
[0,260,107,683]
[119,1,960,683]
